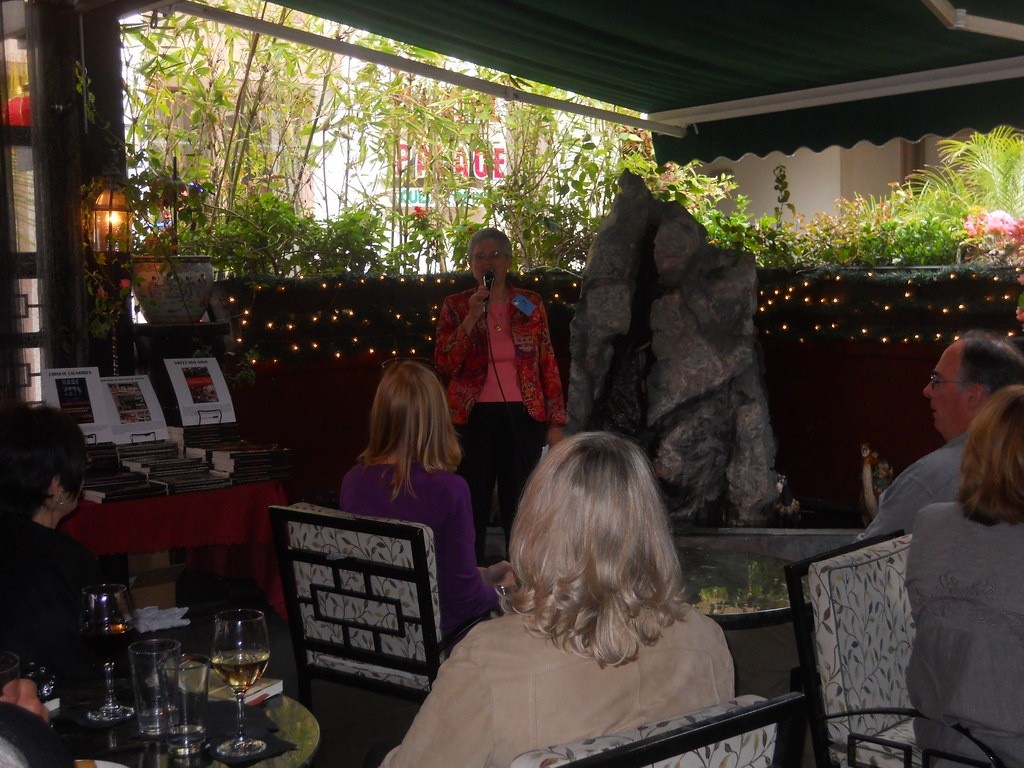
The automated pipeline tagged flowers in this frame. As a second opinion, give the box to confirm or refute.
[962,208,1024,325]
[79,163,214,338]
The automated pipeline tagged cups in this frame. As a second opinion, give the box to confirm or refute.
[128,638,182,735]
[155,654,211,757]
[0,650,21,697]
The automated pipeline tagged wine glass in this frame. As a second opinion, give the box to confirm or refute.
[77,582,135,722]
[211,607,271,757]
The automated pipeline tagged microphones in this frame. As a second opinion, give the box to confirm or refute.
[482,270,495,314]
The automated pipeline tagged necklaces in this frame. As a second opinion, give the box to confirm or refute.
[488,310,505,336]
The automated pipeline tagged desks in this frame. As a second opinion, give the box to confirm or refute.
[670,544,796,630]
[0,658,323,768]
[53,478,292,619]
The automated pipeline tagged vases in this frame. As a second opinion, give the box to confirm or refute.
[120,256,217,327]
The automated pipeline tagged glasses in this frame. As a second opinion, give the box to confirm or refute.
[376,357,440,385]
[472,250,508,261]
[929,372,966,392]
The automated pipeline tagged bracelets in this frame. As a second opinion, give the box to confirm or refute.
[497,585,506,596]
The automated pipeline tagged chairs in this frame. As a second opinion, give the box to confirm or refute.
[269,501,443,709]
[511,689,804,768]
[785,529,1003,768]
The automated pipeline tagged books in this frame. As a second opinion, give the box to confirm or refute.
[81,442,275,506]
[146,657,283,706]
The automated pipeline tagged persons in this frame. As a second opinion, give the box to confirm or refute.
[378,432,735,768]
[1,678,77,768]
[0,401,153,681]
[433,229,569,565]
[905,385,1024,768]
[339,360,517,649]
[846,326,1024,545]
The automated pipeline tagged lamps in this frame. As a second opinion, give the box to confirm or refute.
[81,178,138,268]
